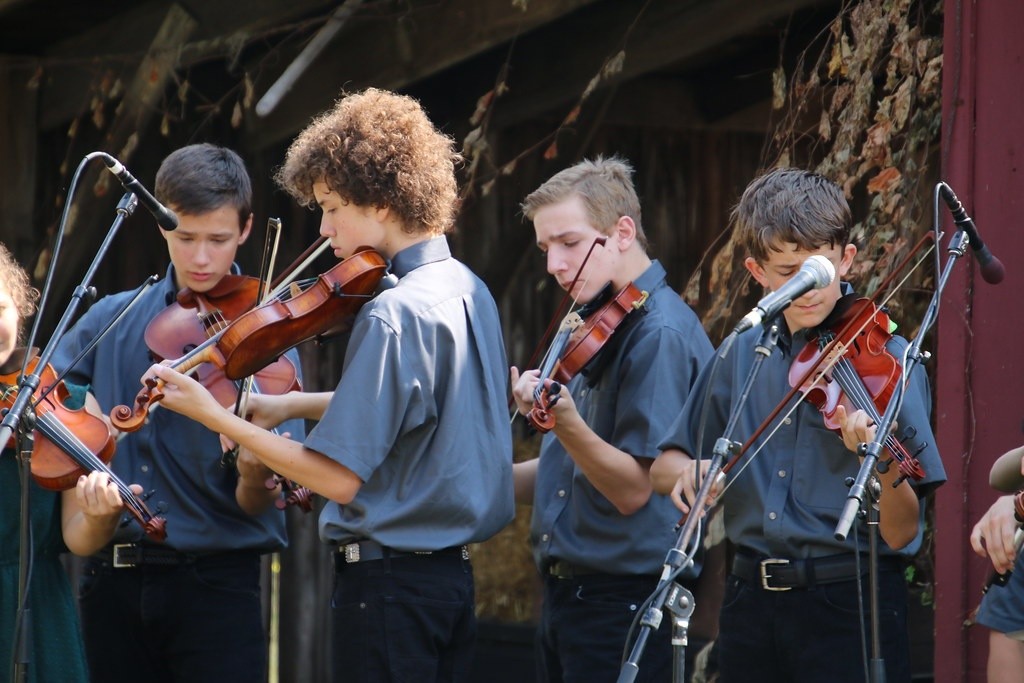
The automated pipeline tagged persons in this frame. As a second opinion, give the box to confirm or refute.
[970,445,1024,683]
[140,87,516,683]
[49,143,306,683]
[0,244,144,683]
[646,169,948,683]
[512,159,715,683]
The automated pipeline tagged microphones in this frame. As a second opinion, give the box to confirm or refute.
[939,181,1005,284]
[99,152,178,231]
[734,255,835,334]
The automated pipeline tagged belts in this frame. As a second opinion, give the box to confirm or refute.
[95,541,230,568]
[331,539,463,567]
[731,552,904,591]
[546,562,606,579]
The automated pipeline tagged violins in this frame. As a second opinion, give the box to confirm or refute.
[1012,490,1024,523]
[108,250,386,433]
[785,296,928,490]
[142,272,320,515]
[524,278,644,435]
[0,354,171,547]
